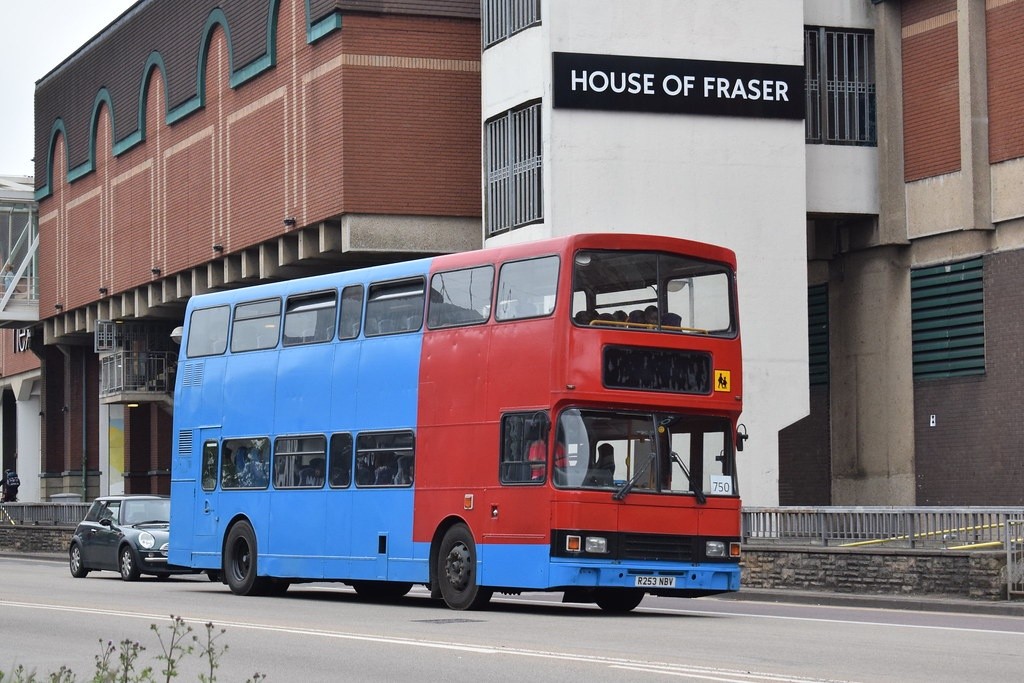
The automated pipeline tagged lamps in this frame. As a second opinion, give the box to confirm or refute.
[99,288,107,295]
[212,246,223,252]
[54,304,63,310]
[283,218,295,226]
[151,269,161,276]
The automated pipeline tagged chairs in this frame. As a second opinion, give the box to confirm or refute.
[297,465,412,485]
[210,313,423,354]
[574,310,683,331]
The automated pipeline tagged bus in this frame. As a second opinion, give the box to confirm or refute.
[168,233,748,610]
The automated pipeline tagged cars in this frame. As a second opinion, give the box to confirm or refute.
[68,491,222,581]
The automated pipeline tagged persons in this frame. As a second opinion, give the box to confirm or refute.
[202,438,414,486]
[575,305,659,330]
[528,422,569,485]
[0,469,20,502]
